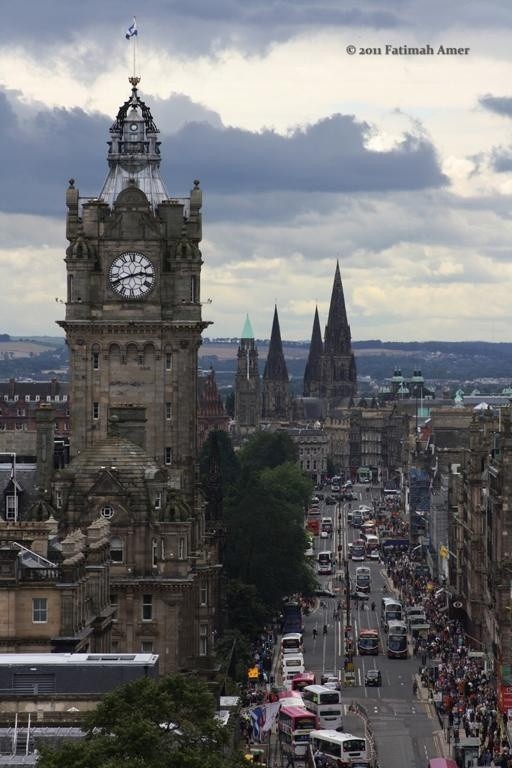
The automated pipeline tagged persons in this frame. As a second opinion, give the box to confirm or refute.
[360,602,365,611]
[372,497,512,768]
[312,628,318,639]
[323,624,327,633]
[287,591,318,616]
[240,620,275,760]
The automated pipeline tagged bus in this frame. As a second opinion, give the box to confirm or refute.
[359,472,369,483]
[347,504,379,561]
[322,517,332,531]
[277,602,369,768]
[304,537,314,558]
[381,596,409,659]
[309,497,320,514]
[317,552,331,574]
[357,566,371,592]
[331,477,340,492]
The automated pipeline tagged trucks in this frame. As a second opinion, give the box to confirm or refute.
[307,518,319,535]
[359,626,378,656]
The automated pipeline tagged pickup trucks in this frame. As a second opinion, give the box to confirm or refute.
[317,590,335,597]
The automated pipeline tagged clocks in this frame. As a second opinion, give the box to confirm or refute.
[104,246,160,302]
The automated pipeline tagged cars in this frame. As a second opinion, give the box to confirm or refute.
[316,494,352,504]
[320,532,330,539]
[350,592,369,601]
[365,669,381,686]
